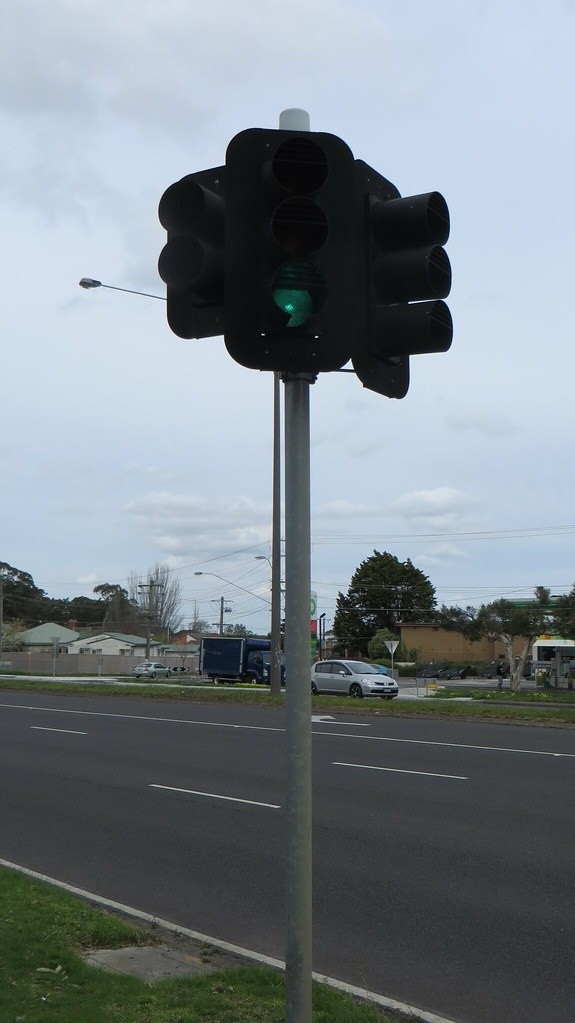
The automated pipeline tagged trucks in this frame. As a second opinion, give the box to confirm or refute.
[199,634,286,687]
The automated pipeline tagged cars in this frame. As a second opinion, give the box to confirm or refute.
[358,663,393,678]
[132,662,171,678]
[310,661,401,700]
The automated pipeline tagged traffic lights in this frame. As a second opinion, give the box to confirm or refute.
[160,128,455,399]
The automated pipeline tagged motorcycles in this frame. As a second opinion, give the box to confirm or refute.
[491,659,508,678]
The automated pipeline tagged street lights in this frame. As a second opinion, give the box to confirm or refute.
[320,612,326,661]
[79,278,282,699]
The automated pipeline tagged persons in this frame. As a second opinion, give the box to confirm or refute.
[543,649,551,661]
[143,657,149,663]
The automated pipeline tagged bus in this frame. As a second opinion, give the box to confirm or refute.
[531,640,575,677]
